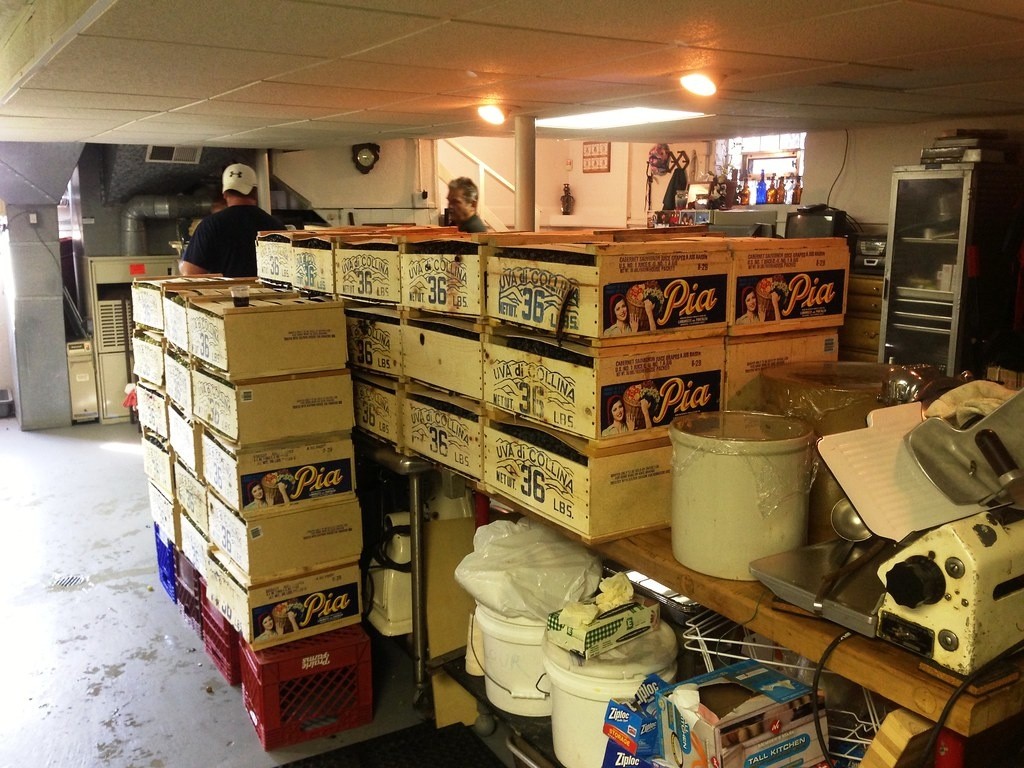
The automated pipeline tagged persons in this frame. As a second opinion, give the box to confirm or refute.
[244,481,274,510]
[255,612,283,642]
[669,213,694,225]
[602,395,636,436]
[181,163,287,278]
[736,286,766,325]
[447,177,487,232]
[604,294,639,336]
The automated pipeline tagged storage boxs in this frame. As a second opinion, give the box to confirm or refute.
[60,220,848,768]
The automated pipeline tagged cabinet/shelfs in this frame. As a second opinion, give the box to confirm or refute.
[837,274,884,364]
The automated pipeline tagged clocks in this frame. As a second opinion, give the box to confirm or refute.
[351,143,381,175]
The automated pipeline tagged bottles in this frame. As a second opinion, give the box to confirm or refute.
[792,176,803,205]
[740,178,750,205]
[776,176,787,204]
[766,177,776,204]
[756,169,767,204]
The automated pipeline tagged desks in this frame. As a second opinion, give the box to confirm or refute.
[458,477,1022,768]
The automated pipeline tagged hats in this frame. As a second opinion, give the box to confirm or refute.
[222,163,258,195]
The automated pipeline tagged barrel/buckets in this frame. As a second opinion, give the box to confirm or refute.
[541,620,677,768]
[670,407,815,582]
[470,598,559,718]
[761,359,895,542]
